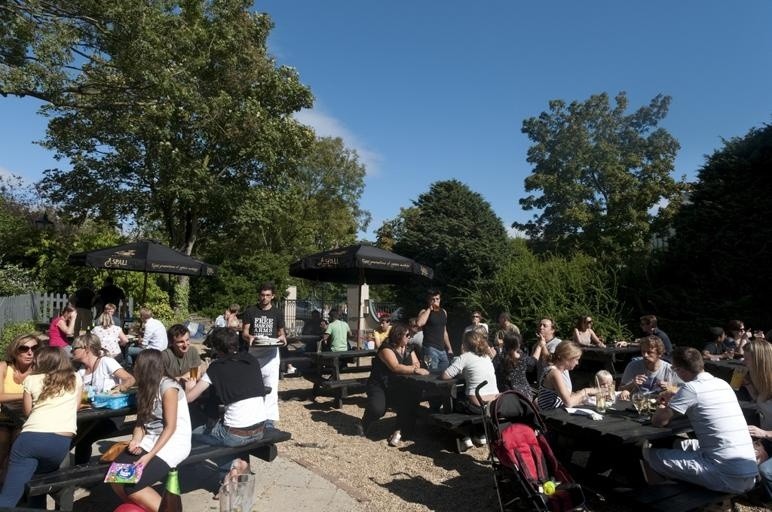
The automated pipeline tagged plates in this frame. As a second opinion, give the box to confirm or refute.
[255,340,270,344]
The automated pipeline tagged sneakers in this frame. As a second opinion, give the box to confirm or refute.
[457,432,487,451]
[388,428,402,447]
[358,419,368,435]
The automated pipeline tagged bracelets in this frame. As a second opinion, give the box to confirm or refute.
[744,381,753,386]
[412,368,417,375]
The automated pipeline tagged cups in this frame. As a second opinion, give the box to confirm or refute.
[607,380,615,401]
[218,474,256,512]
[730,366,749,391]
[631,392,652,417]
[596,391,606,413]
[82,388,89,405]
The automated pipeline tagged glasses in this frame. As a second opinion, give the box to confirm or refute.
[17,344,40,353]
[71,345,83,351]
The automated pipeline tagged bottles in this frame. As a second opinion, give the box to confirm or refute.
[158,467,183,512]
[360,328,376,350]
[86,318,97,335]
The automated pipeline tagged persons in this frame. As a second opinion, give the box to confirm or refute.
[415,288,454,373]
[700,318,766,365]
[0,346,83,512]
[48,303,168,369]
[406,316,424,351]
[738,338,771,501]
[442,311,685,454]
[67,280,98,338]
[213,309,230,329]
[647,345,759,497]
[1,333,40,403]
[354,321,430,449]
[185,325,273,501]
[111,349,192,512]
[161,324,201,389]
[299,304,356,369]
[70,333,137,465]
[96,274,127,318]
[373,312,392,352]
[226,303,241,328]
[241,280,287,421]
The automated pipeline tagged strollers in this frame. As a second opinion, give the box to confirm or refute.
[471,373,607,512]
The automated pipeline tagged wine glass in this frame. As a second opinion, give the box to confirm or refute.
[599,335,619,350]
[424,354,432,372]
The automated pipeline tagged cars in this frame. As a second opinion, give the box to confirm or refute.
[293,298,397,321]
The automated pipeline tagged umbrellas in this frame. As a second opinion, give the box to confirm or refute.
[287,237,434,368]
[67,236,215,308]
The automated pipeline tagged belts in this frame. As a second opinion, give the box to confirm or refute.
[225,424,266,436]
